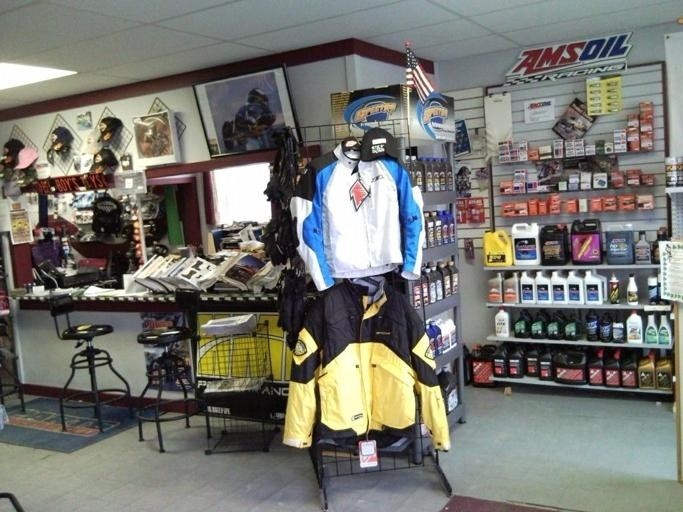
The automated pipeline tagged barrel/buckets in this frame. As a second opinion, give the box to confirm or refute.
[483,230,512,266]
[540,223,567,264]
[511,222,540,266]
[606,224,635,265]
[552,348,588,385]
[470,343,497,388]
[571,219,602,265]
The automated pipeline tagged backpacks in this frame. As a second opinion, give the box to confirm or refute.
[91,196,124,235]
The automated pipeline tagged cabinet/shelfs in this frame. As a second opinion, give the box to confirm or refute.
[482,62,674,400]
[387,131,466,466]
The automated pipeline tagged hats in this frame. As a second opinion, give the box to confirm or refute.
[14,148,39,169]
[0,139,25,165]
[97,116,123,143]
[89,149,119,175]
[360,128,399,161]
[82,131,105,154]
[50,126,74,151]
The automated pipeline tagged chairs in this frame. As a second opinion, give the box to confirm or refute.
[0,319,27,416]
[135,288,210,453]
[48,293,133,433]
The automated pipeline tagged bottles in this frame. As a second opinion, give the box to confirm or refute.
[592,269,609,301]
[566,271,585,305]
[487,272,503,303]
[647,276,658,304]
[432,158,440,191]
[494,307,510,337]
[412,260,458,311]
[519,271,536,304]
[599,312,613,342]
[437,211,449,244]
[430,211,444,246]
[445,158,453,191]
[424,317,458,359]
[513,310,580,340]
[587,348,674,390]
[440,157,445,191]
[665,157,677,186]
[438,367,460,414]
[405,155,425,192]
[652,229,665,264]
[657,273,668,305]
[534,271,552,305]
[658,311,673,345]
[585,309,599,341]
[677,158,683,186]
[627,274,639,306]
[626,309,643,344]
[608,272,619,304]
[59,224,70,256]
[492,342,553,381]
[583,270,603,305]
[442,210,455,244]
[633,230,652,264]
[503,271,520,304]
[424,158,434,192]
[645,311,659,344]
[551,270,569,304]
[612,310,626,344]
[424,212,436,247]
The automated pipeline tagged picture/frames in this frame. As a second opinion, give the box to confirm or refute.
[130,108,181,168]
[191,63,303,158]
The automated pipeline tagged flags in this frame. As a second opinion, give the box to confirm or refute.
[406,46,435,105]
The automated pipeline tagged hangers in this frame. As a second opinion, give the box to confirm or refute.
[342,124,363,156]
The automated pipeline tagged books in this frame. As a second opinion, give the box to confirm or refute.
[200,313,257,336]
[132,252,283,293]
